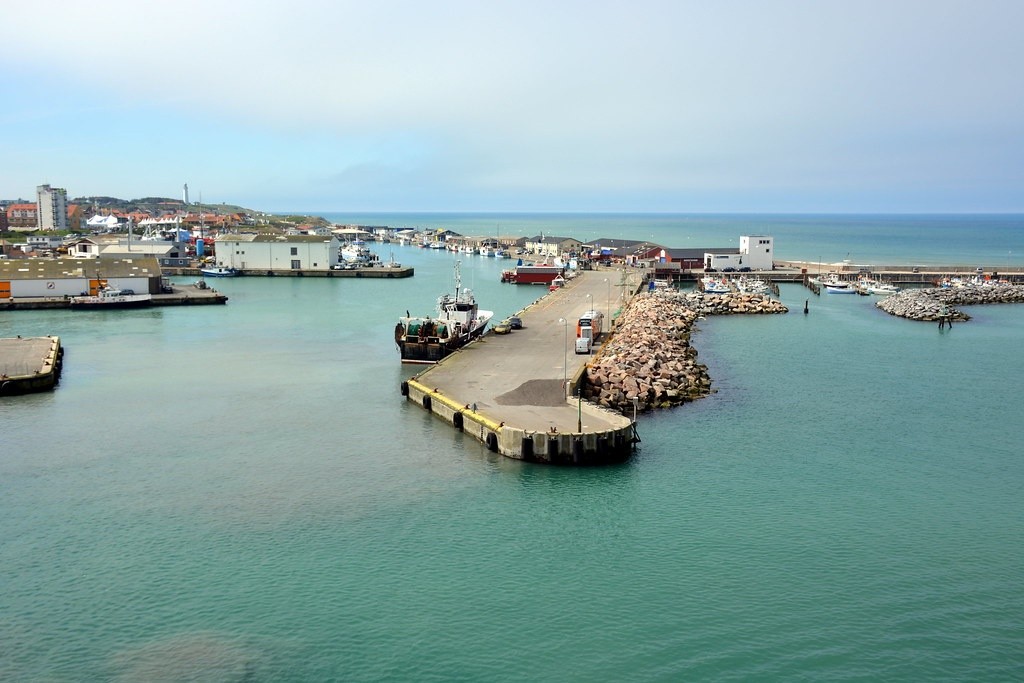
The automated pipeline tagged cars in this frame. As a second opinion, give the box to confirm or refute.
[974,268,983,273]
[704,267,717,273]
[737,267,753,273]
[98,287,135,296]
[494,317,522,334]
[859,268,872,274]
[721,267,735,272]
[333,263,356,270]
[913,268,919,274]
[165,286,174,293]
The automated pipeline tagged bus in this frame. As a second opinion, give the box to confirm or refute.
[575,310,604,354]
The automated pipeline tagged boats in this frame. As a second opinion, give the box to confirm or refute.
[418,238,511,259]
[936,273,1009,288]
[699,274,770,295]
[808,272,900,294]
[200,263,237,278]
[394,260,494,364]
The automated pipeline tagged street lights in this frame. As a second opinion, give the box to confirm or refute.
[587,293,594,356]
[604,277,611,332]
[559,317,567,400]
